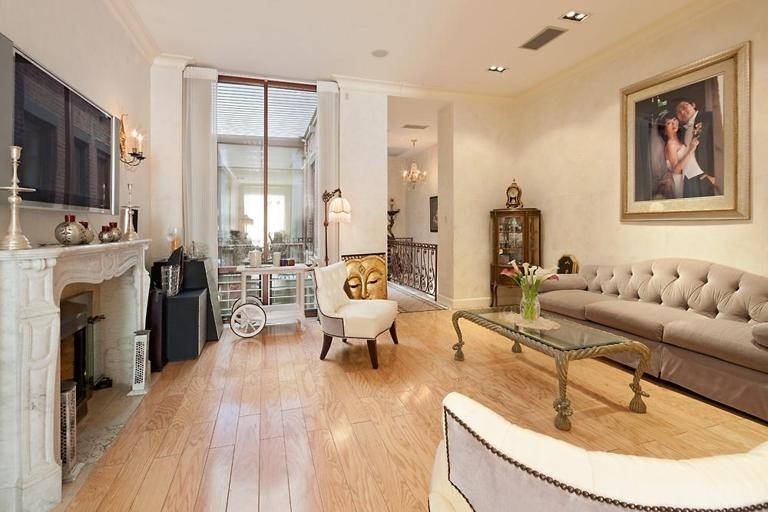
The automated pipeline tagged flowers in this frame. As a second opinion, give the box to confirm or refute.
[500,260,560,323]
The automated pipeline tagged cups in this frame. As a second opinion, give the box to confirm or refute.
[272,252,281,266]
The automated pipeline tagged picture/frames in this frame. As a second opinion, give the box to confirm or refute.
[611,39,756,225]
[430,195,439,233]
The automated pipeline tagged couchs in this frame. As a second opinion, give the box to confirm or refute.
[521,258,764,420]
[428,389,763,512]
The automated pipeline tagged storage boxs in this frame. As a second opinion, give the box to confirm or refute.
[491,208,541,311]
[162,287,208,362]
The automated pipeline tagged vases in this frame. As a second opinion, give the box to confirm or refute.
[520,291,542,325]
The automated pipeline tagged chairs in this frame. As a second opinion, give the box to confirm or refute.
[314,261,400,370]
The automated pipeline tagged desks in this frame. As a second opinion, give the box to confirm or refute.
[237,262,311,338]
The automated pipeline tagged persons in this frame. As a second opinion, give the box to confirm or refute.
[673,98,714,197]
[657,112,699,198]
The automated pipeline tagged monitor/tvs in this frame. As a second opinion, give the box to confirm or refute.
[11,45,117,216]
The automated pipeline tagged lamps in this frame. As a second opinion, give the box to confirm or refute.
[402,139,428,191]
[322,192,351,263]
[123,126,145,166]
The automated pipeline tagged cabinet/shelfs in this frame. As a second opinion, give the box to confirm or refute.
[0,238,155,510]
